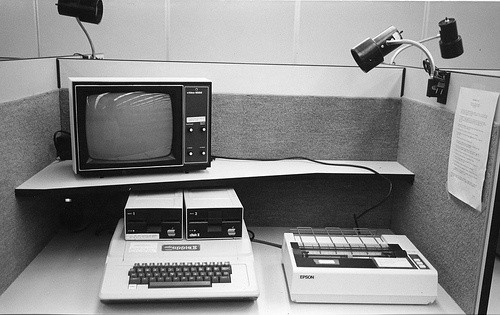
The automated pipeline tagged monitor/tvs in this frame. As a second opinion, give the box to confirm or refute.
[68,75,212,177]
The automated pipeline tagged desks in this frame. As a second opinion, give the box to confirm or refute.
[1,223,467,314]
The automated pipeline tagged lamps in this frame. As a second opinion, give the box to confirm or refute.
[350,26,450,105]
[55,0,103,60]
[390,17,464,66]
